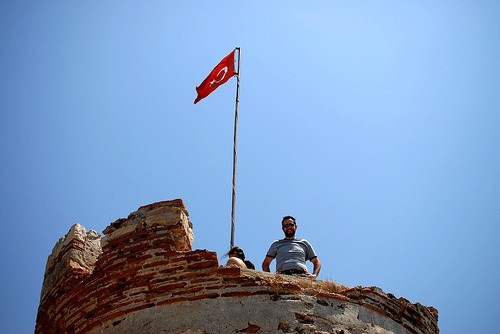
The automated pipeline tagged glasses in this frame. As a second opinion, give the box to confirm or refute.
[283,224,295,227]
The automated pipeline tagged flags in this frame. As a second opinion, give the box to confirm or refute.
[194,50,236,104]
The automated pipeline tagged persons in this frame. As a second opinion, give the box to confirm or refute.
[228,246,255,270]
[262,216,321,279]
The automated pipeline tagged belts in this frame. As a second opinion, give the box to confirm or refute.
[280,269,303,275]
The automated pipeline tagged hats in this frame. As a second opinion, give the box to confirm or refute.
[228,246,244,255]
